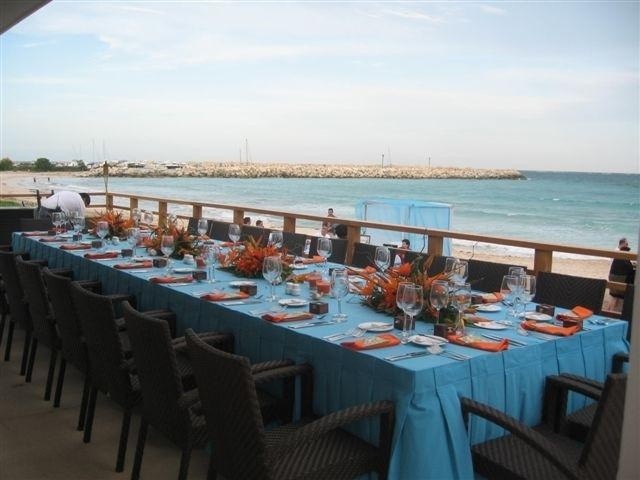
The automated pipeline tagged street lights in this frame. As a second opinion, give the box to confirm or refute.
[95,160,120,195]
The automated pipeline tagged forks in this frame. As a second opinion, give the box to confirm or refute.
[323,327,368,343]
[586,316,623,327]
[426,343,474,361]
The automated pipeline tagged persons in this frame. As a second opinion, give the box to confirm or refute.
[400,239,412,250]
[244,216,252,225]
[319,208,348,239]
[608,246,635,299]
[610,238,636,270]
[36,191,90,221]
[255,220,264,228]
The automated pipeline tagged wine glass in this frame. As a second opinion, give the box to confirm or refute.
[430,258,472,330]
[375,245,390,275]
[396,282,423,345]
[268,228,284,250]
[496,266,538,336]
[316,236,333,275]
[328,268,350,323]
[52,211,241,285]
[262,253,286,304]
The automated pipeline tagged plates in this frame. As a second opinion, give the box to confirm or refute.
[473,320,513,330]
[356,321,395,333]
[519,311,553,322]
[409,334,449,345]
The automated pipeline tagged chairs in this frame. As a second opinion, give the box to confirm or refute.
[0,218,637,478]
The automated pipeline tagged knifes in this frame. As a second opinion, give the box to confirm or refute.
[480,332,525,346]
[288,320,330,330]
[222,300,264,306]
[384,350,430,362]
